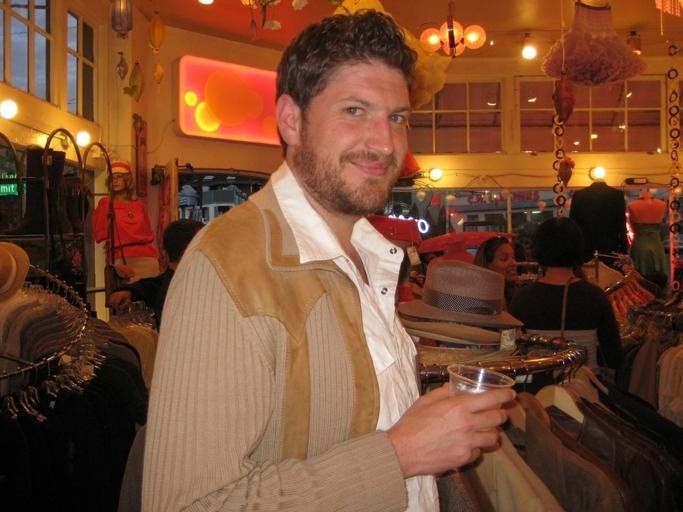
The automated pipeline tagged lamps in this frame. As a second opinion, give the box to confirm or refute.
[418,0,487,60]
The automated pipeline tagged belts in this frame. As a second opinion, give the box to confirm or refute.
[113,240,148,247]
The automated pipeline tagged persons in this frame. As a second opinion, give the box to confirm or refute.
[470,234,520,278]
[408,250,446,289]
[626,188,669,279]
[395,254,438,349]
[565,176,629,278]
[57,243,84,282]
[137,5,520,512]
[148,218,206,334]
[505,215,625,392]
[104,271,166,313]
[89,160,160,267]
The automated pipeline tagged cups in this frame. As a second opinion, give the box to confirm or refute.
[446,363,514,407]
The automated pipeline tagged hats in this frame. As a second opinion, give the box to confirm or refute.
[1,241,30,303]
[397,258,523,346]
[106,160,132,175]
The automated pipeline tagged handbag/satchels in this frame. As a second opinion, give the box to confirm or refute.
[104,263,134,294]
[526,328,599,373]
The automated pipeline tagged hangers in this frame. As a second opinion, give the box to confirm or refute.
[604,254,683,361]
[419,347,683,512]
[0,264,139,444]
